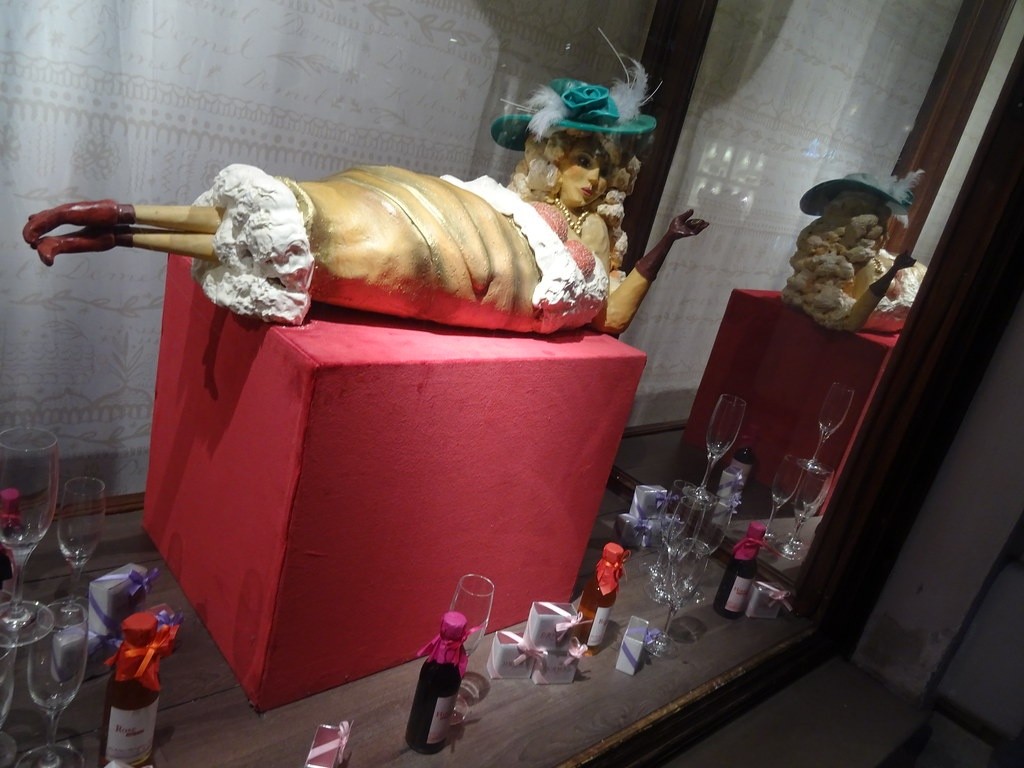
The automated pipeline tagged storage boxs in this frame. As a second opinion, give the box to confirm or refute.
[304,720,354,768]
[711,465,744,524]
[745,581,792,618]
[486,602,588,685]
[616,615,662,676]
[614,485,680,550]
[51,563,183,685]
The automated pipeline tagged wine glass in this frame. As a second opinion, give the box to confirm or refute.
[643,393,747,659]
[449,572,494,728]
[0,426,108,767]
[762,382,855,562]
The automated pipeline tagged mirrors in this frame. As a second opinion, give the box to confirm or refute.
[609,0,1024,595]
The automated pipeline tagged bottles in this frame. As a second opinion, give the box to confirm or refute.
[573,544,624,656]
[99,612,158,767]
[0,489,20,598]
[713,522,767,621]
[404,610,468,756]
